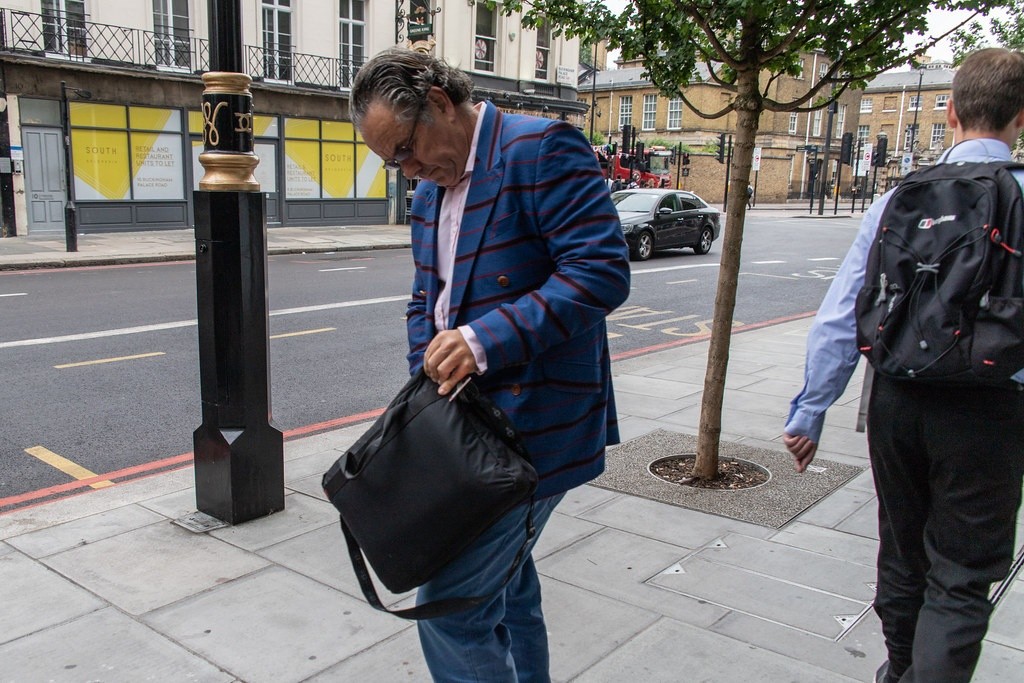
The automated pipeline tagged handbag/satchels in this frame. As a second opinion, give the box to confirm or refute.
[323,365,541,621]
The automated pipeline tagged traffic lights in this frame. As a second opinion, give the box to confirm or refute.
[670,145,676,164]
[871,151,879,167]
[683,153,690,166]
[716,133,725,164]
[620,125,631,154]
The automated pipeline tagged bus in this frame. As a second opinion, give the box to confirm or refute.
[593,144,673,189]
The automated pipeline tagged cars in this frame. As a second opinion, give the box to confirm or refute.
[611,188,723,262]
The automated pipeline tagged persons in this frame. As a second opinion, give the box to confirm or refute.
[605,175,645,192]
[782,48,1024,683]
[349,46,631,683]
[747,182,752,210]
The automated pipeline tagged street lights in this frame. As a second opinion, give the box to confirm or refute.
[909,65,927,154]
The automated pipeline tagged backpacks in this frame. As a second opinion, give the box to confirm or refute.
[855,160,1024,380]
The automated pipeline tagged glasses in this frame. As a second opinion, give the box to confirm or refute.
[381,87,428,172]
